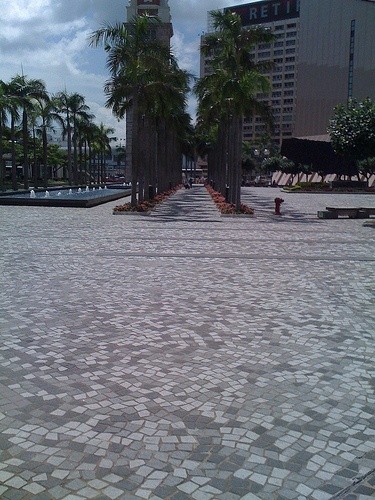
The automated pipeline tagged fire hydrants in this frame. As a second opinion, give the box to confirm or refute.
[274,197,285,213]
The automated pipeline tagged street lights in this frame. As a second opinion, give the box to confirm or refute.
[254,148,270,185]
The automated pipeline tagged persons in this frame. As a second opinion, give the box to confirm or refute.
[182,170,277,192]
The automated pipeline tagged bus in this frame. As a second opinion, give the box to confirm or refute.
[182,168,207,180]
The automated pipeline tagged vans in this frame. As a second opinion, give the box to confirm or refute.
[254,175,267,184]
